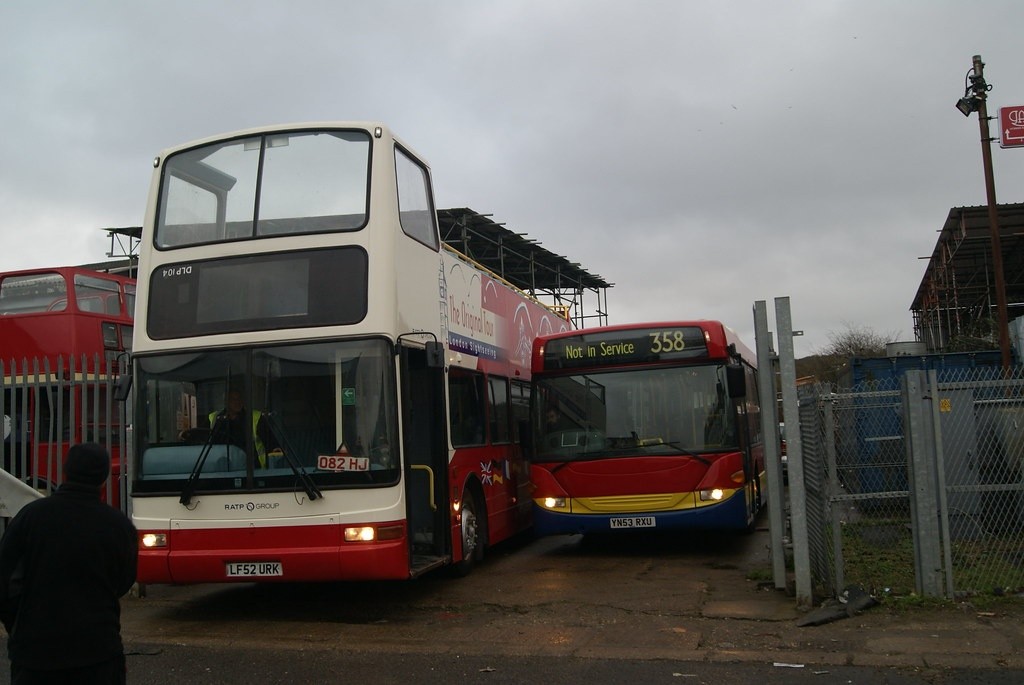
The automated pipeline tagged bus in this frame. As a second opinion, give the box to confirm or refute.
[0,268,135,506]
[127,121,569,589]
[524,320,774,539]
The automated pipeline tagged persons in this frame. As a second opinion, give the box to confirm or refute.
[1,442,140,685]
[176,380,284,471]
[541,407,568,453]
